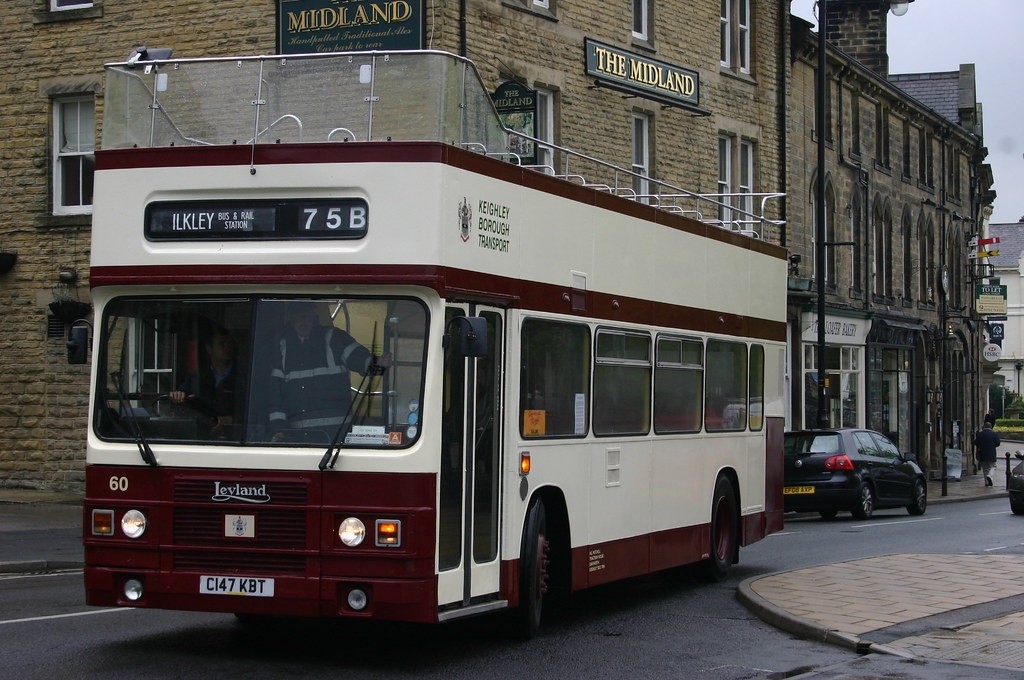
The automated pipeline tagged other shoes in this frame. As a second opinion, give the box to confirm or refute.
[985,476,993,486]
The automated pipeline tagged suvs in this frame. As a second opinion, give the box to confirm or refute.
[782,427,929,517]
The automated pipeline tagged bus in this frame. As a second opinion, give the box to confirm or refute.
[63,47,793,638]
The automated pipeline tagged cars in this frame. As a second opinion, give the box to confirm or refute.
[1008,449,1024,516]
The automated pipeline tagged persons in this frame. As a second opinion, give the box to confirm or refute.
[983,408,996,429]
[975,429,981,470]
[974,422,1001,486]
[267,298,393,444]
[169,327,254,424]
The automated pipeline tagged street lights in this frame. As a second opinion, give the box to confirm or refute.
[813,0,915,429]
